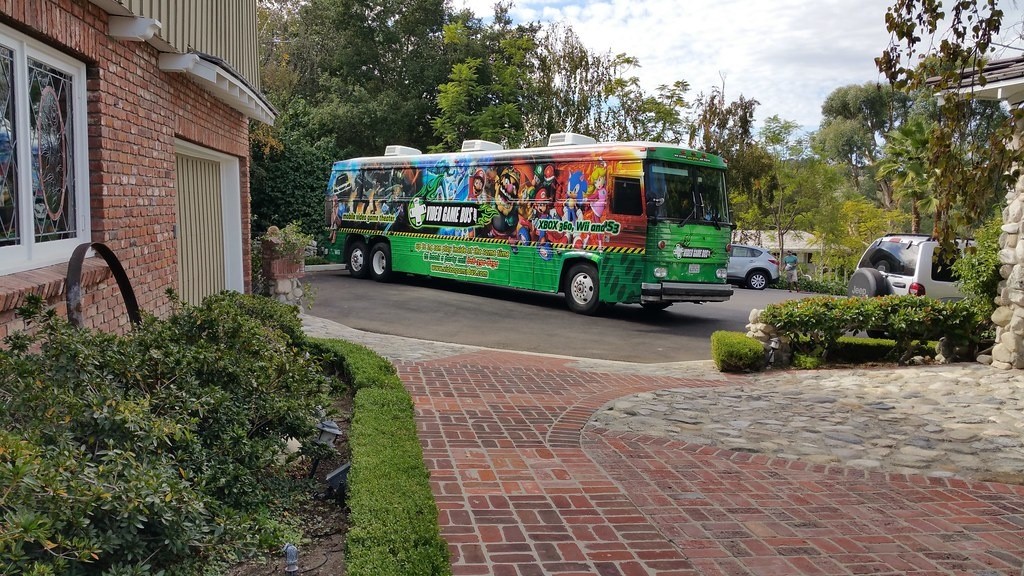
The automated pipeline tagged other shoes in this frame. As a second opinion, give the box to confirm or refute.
[797,290,799,293]
[789,291,791,292]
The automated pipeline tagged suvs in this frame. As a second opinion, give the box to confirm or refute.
[727,243,780,290]
[848,233,978,339]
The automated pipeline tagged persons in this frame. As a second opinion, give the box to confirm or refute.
[779,250,800,293]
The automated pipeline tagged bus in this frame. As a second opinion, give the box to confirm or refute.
[325,133,737,315]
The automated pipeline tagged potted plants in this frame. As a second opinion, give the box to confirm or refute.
[256,220,314,280]
